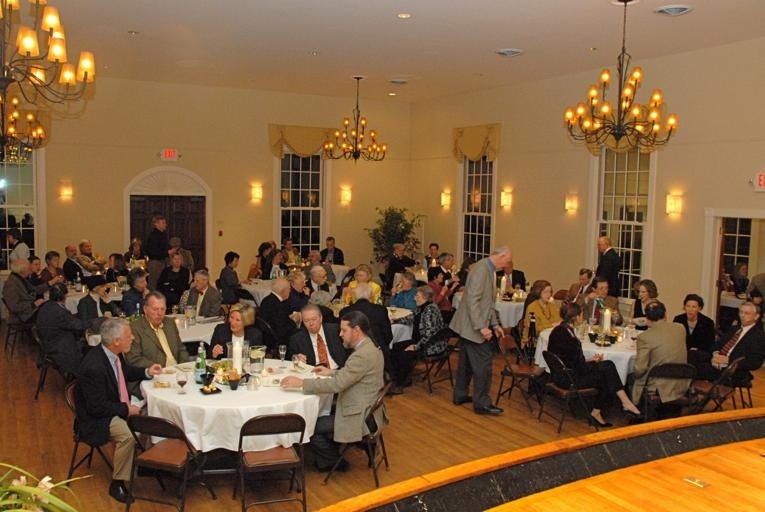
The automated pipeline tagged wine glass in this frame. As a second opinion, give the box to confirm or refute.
[176,342,307,396]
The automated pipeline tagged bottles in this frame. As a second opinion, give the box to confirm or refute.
[135,303,141,320]
[75,271,82,292]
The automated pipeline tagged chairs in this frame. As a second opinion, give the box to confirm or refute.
[2,269,756,512]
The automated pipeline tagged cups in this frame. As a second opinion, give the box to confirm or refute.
[495,283,526,301]
[576,318,639,348]
[390,305,397,314]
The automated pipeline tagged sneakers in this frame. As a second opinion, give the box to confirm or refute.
[313,460,349,474]
[365,442,379,468]
[137,466,162,477]
[385,375,414,396]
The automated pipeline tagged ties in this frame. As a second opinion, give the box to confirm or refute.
[578,286,584,296]
[115,356,130,406]
[506,274,512,289]
[593,299,602,325]
[718,327,744,355]
[316,332,331,370]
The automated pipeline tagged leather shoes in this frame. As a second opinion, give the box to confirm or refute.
[453,396,473,405]
[474,404,504,415]
[109,482,135,504]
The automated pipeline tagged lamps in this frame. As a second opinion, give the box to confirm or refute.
[440,191,451,207]
[563,0,679,155]
[323,76,388,163]
[0,91,48,166]
[251,186,263,199]
[1,0,100,105]
[564,191,578,212]
[500,191,513,207]
[665,192,683,215]
[58,180,71,196]
[341,189,352,202]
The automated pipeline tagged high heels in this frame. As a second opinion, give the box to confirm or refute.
[588,413,613,428]
[622,407,645,418]
